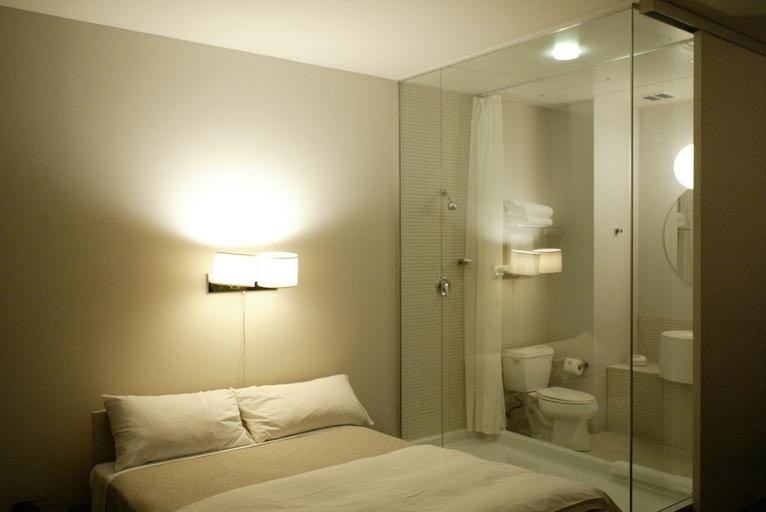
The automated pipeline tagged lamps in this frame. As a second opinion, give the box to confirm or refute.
[492,247,564,280]
[206,251,300,293]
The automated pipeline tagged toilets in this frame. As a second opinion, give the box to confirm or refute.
[502,343,599,452]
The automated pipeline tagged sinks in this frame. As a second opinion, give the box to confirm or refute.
[658,329,694,387]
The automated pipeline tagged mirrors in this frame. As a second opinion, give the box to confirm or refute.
[664,189,694,286]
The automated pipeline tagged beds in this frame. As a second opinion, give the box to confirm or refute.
[85,371,624,511]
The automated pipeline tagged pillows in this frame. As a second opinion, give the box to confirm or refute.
[229,372,375,444]
[97,388,257,473]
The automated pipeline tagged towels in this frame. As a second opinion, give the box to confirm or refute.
[505,198,553,216]
[505,216,554,226]
